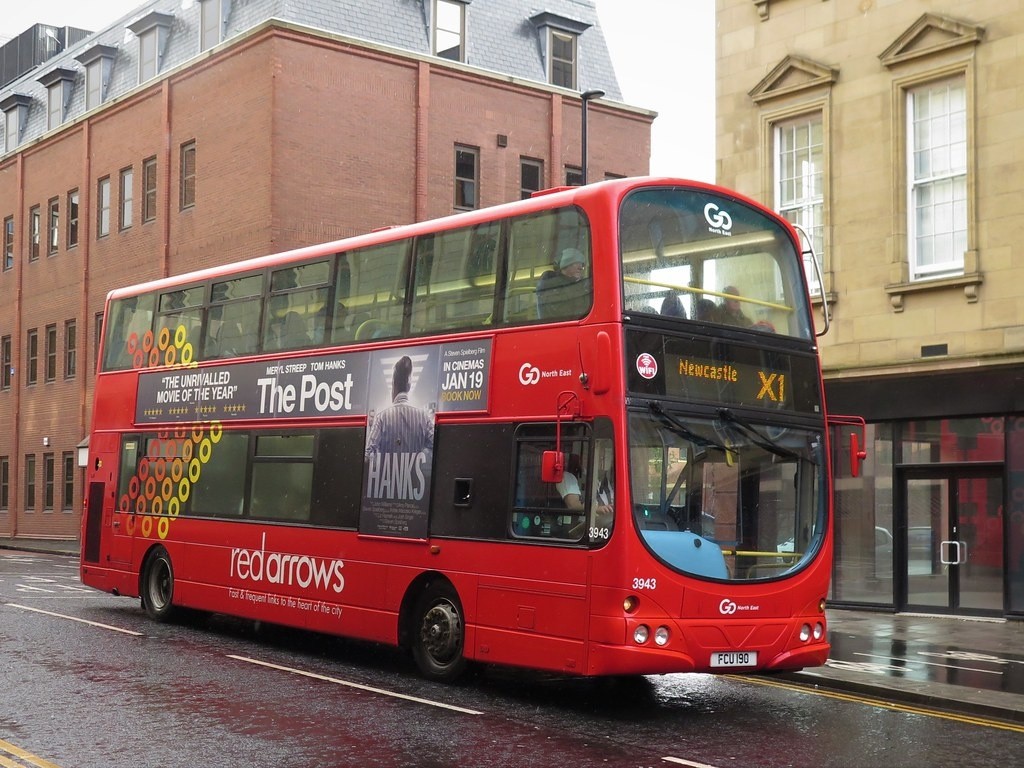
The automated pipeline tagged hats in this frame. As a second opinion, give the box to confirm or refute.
[559,247,586,271]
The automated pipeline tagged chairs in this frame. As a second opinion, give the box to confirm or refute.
[118,270,720,340]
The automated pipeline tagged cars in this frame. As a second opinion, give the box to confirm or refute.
[832,522,934,566]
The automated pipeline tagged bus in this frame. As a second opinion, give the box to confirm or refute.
[77,176,871,688]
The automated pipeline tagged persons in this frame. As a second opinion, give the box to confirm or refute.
[365,355,435,467]
[536,439,614,540]
[534,248,757,330]
[314,301,354,348]
